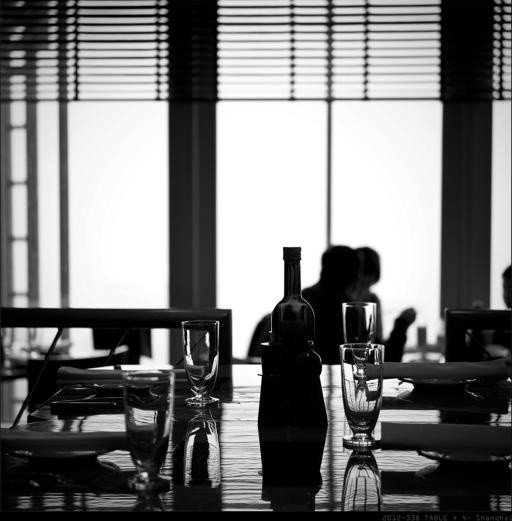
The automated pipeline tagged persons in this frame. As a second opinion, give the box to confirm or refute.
[472,268,512,364]
[248,244,359,367]
[350,246,416,365]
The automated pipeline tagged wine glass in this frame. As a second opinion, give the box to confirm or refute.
[341,445,382,512]
[181,319,220,405]
[341,301,377,379]
[339,342,386,446]
[124,372,175,492]
[183,405,221,488]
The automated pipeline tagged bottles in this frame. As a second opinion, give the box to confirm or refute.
[272,247,316,339]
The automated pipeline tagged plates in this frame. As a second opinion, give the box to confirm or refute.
[14,448,110,459]
[81,383,125,390]
[417,446,511,462]
[398,376,480,385]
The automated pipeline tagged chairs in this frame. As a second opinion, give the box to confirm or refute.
[0,308,232,365]
[446,309,512,363]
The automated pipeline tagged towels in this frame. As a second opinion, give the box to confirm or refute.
[363,358,511,379]
[381,422,511,455]
[0,429,134,450]
[57,366,206,383]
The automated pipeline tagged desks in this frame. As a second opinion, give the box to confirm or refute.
[0,354,505,513]
